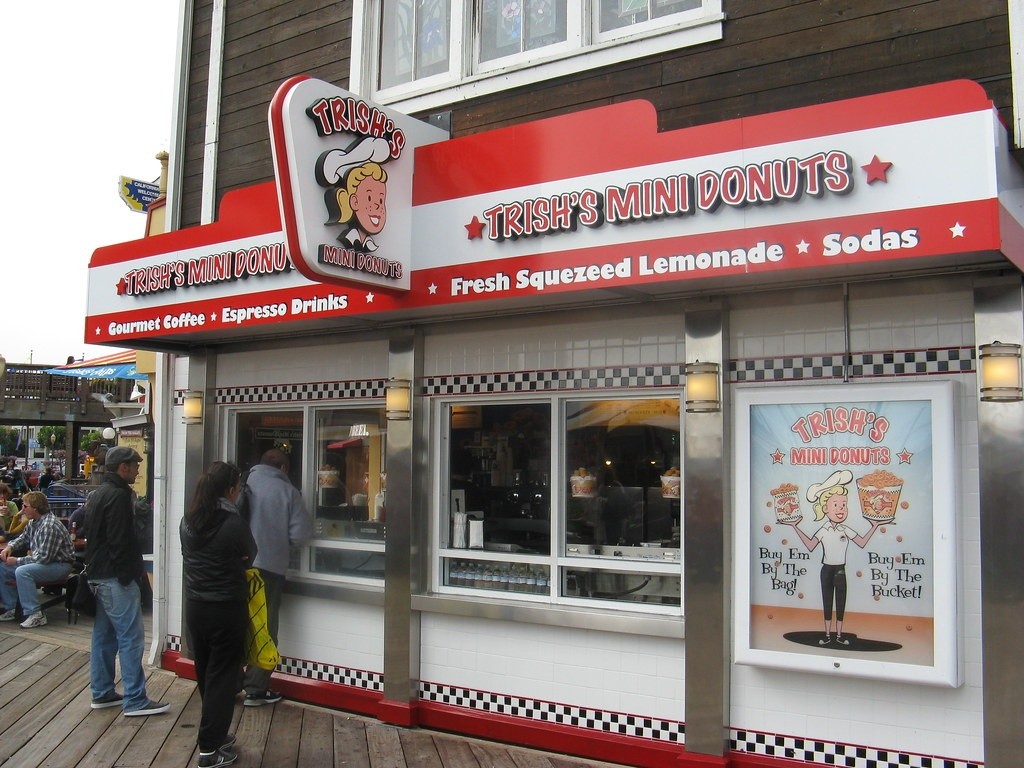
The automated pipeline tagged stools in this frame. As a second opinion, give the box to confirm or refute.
[14,573,79,624]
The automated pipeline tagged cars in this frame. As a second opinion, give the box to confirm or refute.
[28,471,45,486]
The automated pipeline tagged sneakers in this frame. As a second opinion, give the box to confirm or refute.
[19,611,48,627]
[243,689,282,706]
[91,693,123,708]
[197,749,238,768]
[124,700,171,715]
[0,609,15,620]
[219,735,237,750]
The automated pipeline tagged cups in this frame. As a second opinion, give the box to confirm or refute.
[453,522,467,549]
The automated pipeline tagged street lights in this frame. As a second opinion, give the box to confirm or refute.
[102,428,115,448]
[50,434,57,469]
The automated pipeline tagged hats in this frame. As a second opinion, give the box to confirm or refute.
[105,446,143,466]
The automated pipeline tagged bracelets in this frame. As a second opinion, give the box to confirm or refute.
[17,558,21,565]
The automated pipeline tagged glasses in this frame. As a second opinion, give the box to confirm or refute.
[23,505,32,508]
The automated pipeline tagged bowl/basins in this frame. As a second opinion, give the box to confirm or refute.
[319,470,340,490]
[660,476,680,499]
[570,476,598,498]
[0,505,7,517]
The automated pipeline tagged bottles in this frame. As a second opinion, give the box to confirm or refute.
[449,562,550,596]
[70,521,77,541]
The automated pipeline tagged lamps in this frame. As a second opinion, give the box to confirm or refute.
[684,359,721,413]
[385,377,412,421]
[979,341,1024,403]
[182,390,204,425]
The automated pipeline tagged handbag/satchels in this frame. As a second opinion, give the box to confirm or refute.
[243,568,281,670]
[234,471,251,522]
[69,574,97,617]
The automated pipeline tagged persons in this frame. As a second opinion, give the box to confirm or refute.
[242,445,313,704]
[450,467,478,519]
[68,490,95,530]
[1,491,77,628]
[84,445,171,716]
[0,457,54,542]
[178,460,257,768]
